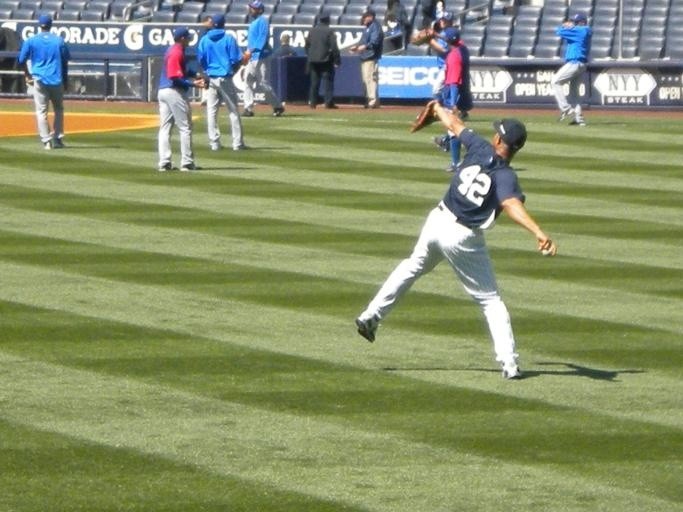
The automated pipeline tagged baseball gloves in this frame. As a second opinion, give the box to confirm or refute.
[413,29,438,44]
[408,97,440,134]
[242,51,251,65]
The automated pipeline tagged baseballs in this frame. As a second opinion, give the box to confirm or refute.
[541,243,552,256]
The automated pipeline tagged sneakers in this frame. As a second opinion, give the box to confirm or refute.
[445,161,461,172]
[356,309,380,342]
[54,139,64,148]
[558,107,575,121]
[43,142,52,150]
[500,364,523,379]
[432,133,449,151]
[569,119,586,125]
[273,105,284,116]
[181,163,201,171]
[158,163,178,171]
[239,108,252,116]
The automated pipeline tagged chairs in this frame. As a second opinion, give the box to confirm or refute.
[391,0,682,62]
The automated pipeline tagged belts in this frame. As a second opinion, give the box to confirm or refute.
[437,204,472,229]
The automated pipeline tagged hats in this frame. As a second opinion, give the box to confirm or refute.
[173,0,264,40]
[570,13,588,22]
[38,14,51,26]
[493,118,527,151]
[438,11,453,21]
[446,27,460,43]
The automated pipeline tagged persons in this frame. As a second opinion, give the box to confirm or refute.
[204,16,213,24]
[272,33,296,55]
[17,13,72,150]
[385,1,407,37]
[552,13,593,127]
[198,13,248,150]
[355,99,556,379]
[349,10,383,108]
[156,26,207,173]
[304,13,341,108]
[415,1,474,173]
[238,0,284,117]
